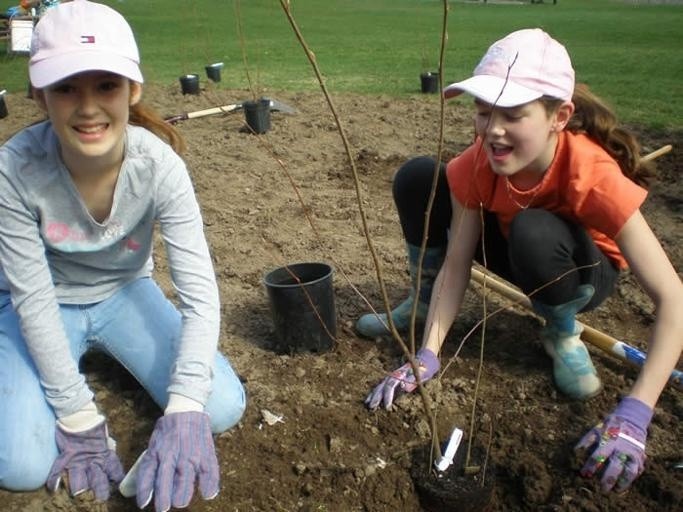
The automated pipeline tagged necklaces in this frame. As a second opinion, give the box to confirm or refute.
[506,176,547,212]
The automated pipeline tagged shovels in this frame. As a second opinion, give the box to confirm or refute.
[163,96,298,124]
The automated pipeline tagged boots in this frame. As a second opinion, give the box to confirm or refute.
[531,283,602,403]
[356,243,447,339]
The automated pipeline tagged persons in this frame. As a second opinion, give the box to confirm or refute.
[0,1,247,512]
[355,28,682,495]
[21,1,60,99]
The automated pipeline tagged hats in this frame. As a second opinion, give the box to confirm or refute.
[440,27,576,108]
[28,1,145,90]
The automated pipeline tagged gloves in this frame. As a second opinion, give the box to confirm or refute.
[119,411,220,512]
[47,417,126,503]
[365,346,443,410]
[570,397,654,494]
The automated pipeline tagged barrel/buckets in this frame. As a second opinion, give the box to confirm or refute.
[420,56,438,94]
[205,65,221,83]
[0,89,9,121]
[8,8,33,52]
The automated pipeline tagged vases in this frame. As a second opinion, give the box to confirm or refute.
[419,71,440,95]
[204,63,222,84]
